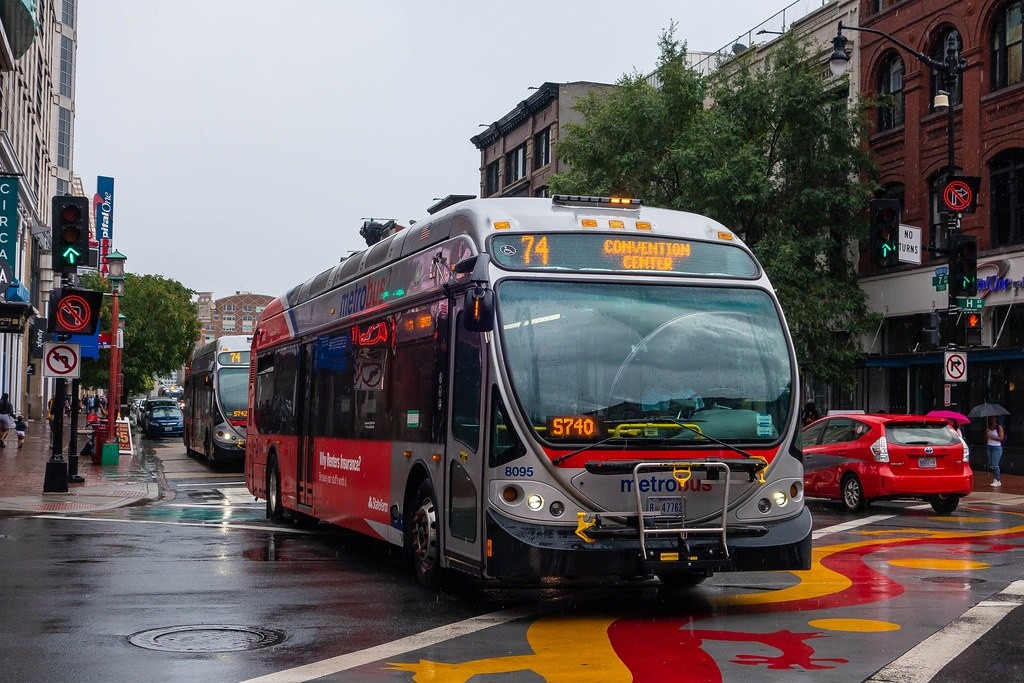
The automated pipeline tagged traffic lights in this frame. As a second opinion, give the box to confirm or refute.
[962,314,981,346]
[869,199,899,268]
[52,195,89,274]
[947,234,978,297]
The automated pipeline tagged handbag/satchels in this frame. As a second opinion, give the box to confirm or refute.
[47,411,51,420]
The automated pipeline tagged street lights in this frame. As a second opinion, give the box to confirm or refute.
[828,20,983,413]
[101,249,127,468]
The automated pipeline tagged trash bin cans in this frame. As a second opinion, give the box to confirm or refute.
[91,420,119,464]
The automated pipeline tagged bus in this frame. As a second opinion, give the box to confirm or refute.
[182,334,255,463]
[244,193,814,599]
[158,386,184,411]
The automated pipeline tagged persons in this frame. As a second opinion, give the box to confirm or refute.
[987,415,1004,487]
[0,393,17,448]
[203,421,211,456]
[948,418,962,437]
[876,410,885,414]
[64,394,108,417]
[158,388,166,396]
[642,352,704,417]
[762,355,791,436]
[48,394,55,448]
[853,425,864,441]
[254,399,292,435]
[801,399,819,427]
[14,415,28,448]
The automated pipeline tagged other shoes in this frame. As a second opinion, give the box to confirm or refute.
[990,479,1002,487]
[0,440,6,448]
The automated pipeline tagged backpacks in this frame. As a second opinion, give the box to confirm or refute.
[996,424,1007,446]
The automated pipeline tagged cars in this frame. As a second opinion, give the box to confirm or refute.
[802,413,974,514]
[132,399,182,436]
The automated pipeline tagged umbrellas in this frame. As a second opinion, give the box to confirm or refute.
[926,411,970,426]
[968,402,1010,430]
